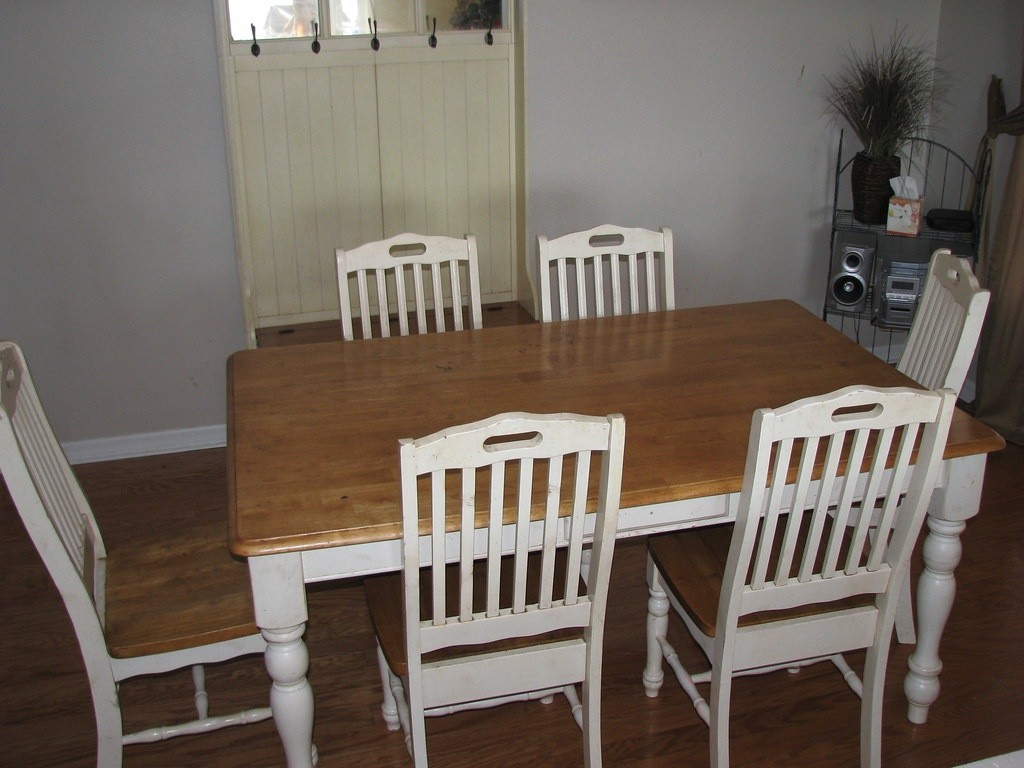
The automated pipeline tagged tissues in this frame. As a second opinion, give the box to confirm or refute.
[885,175,926,238]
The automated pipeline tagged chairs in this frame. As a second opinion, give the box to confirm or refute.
[786,249,992,675]
[536,224,674,322]
[365,411,626,768]
[335,233,484,340]
[0,342,326,768]
[640,384,955,768]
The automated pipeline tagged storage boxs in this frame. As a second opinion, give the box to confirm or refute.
[885,193,927,237]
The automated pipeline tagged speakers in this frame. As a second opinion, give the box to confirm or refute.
[931,240,976,279]
[825,231,876,313]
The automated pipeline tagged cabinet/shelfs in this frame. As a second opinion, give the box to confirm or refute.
[824,129,992,367]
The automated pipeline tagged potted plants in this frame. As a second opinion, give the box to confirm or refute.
[819,25,957,224]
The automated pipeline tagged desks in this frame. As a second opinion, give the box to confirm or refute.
[226,300,1004,768]
